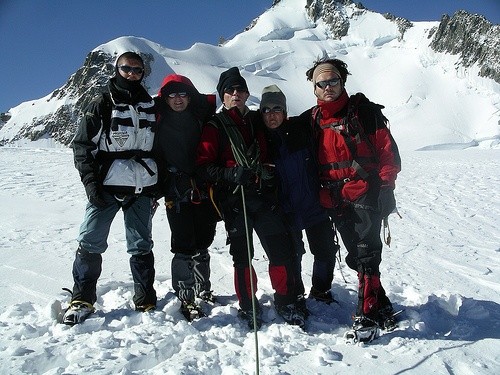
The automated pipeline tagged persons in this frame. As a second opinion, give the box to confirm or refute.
[62,51,159,326]
[297,58,402,344]
[151,74,216,321]
[193,65,305,329]
[258,84,334,315]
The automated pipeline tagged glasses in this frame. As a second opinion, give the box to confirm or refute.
[261,106,283,113]
[316,78,339,89]
[117,66,144,74]
[166,89,188,98]
[223,86,248,93]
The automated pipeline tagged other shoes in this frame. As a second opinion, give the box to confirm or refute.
[181,302,208,322]
[197,290,216,303]
[308,287,339,305]
[352,315,378,344]
[238,306,263,331]
[273,294,305,328]
[62,303,92,325]
[135,304,157,312]
[377,297,396,330]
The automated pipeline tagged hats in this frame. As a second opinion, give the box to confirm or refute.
[217,67,248,103]
[260,84,286,112]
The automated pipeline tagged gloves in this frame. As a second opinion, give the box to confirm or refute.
[227,165,256,184]
[175,174,202,202]
[85,177,110,208]
[378,189,396,220]
